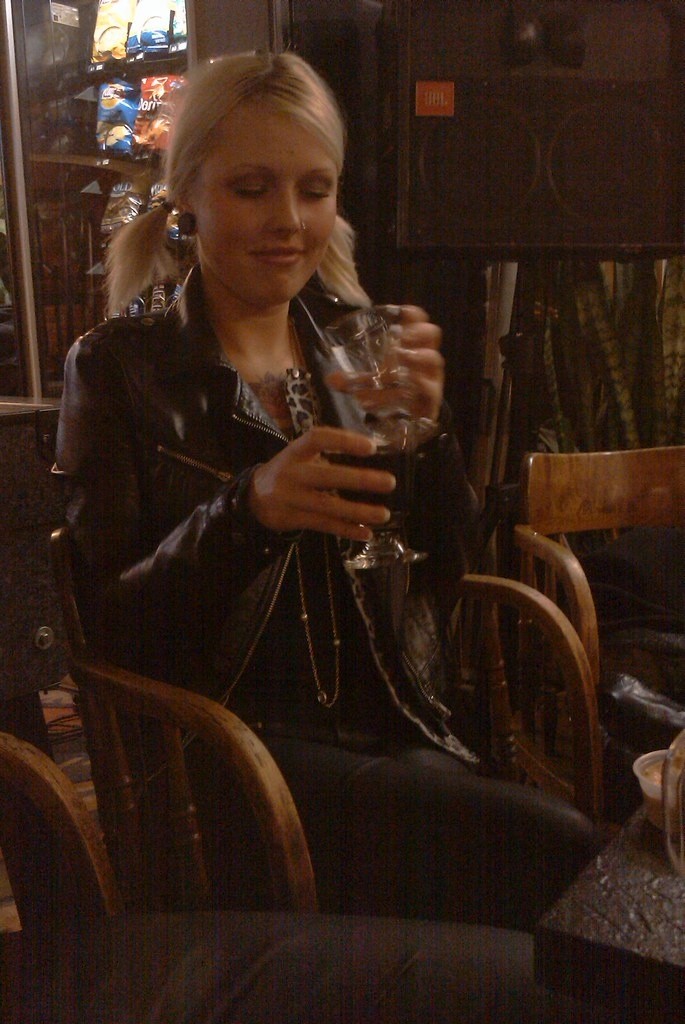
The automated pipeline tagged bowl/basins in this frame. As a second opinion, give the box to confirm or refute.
[632,748,668,829]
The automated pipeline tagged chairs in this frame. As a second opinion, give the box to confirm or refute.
[1,442,685,1023]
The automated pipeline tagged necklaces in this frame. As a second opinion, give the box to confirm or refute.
[289,320,342,710]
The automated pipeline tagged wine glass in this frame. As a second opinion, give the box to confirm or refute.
[330,307,440,457]
[327,441,428,570]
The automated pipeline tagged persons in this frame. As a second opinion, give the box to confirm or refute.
[1,911,550,1024]
[44,47,600,934]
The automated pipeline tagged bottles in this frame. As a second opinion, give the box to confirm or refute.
[661,731,685,876]
